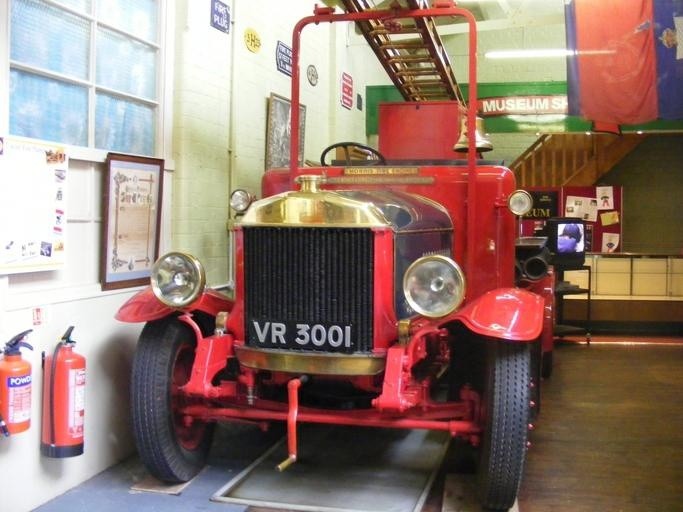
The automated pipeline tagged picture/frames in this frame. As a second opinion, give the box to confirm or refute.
[97,153,167,292]
[264,91,306,169]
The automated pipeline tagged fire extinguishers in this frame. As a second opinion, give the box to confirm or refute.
[41,325,86,458]
[0,329,34,436]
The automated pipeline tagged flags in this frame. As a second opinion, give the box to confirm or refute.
[562,0,682,124]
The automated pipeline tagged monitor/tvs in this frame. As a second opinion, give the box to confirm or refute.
[534,217,586,269]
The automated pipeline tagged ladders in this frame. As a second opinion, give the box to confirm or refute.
[338,0,464,101]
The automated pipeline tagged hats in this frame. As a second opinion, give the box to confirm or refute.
[558,238,576,252]
[559,224,580,236]
